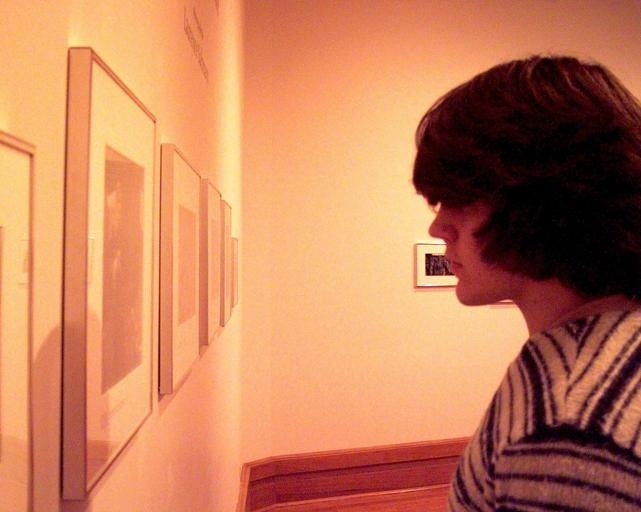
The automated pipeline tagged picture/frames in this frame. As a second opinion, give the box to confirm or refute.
[200,179,222,347]
[413,243,460,288]
[160,141,201,395]
[62,45,161,505]
[0,129,44,511]
[221,201,235,326]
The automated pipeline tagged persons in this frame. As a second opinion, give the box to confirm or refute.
[411,52,641,512]
